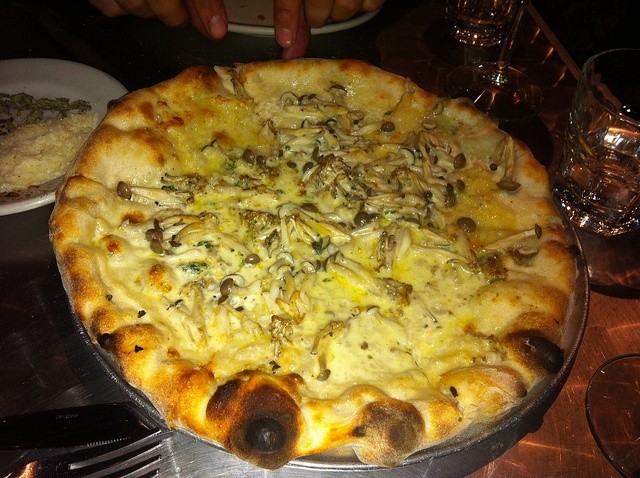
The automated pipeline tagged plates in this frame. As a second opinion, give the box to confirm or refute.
[0,57,129,217]
[224,0,382,39]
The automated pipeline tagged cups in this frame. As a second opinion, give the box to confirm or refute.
[549,47,640,239]
[444,0,518,48]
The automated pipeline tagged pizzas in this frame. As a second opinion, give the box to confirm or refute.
[48,56,583,474]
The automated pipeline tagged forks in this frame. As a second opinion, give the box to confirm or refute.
[0,429,177,478]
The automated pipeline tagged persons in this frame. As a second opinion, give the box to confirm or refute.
[90,1,379,60]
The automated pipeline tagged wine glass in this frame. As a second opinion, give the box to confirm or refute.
[444,0,543,124]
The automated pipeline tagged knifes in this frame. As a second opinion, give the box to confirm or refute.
[0,401,148,450]
